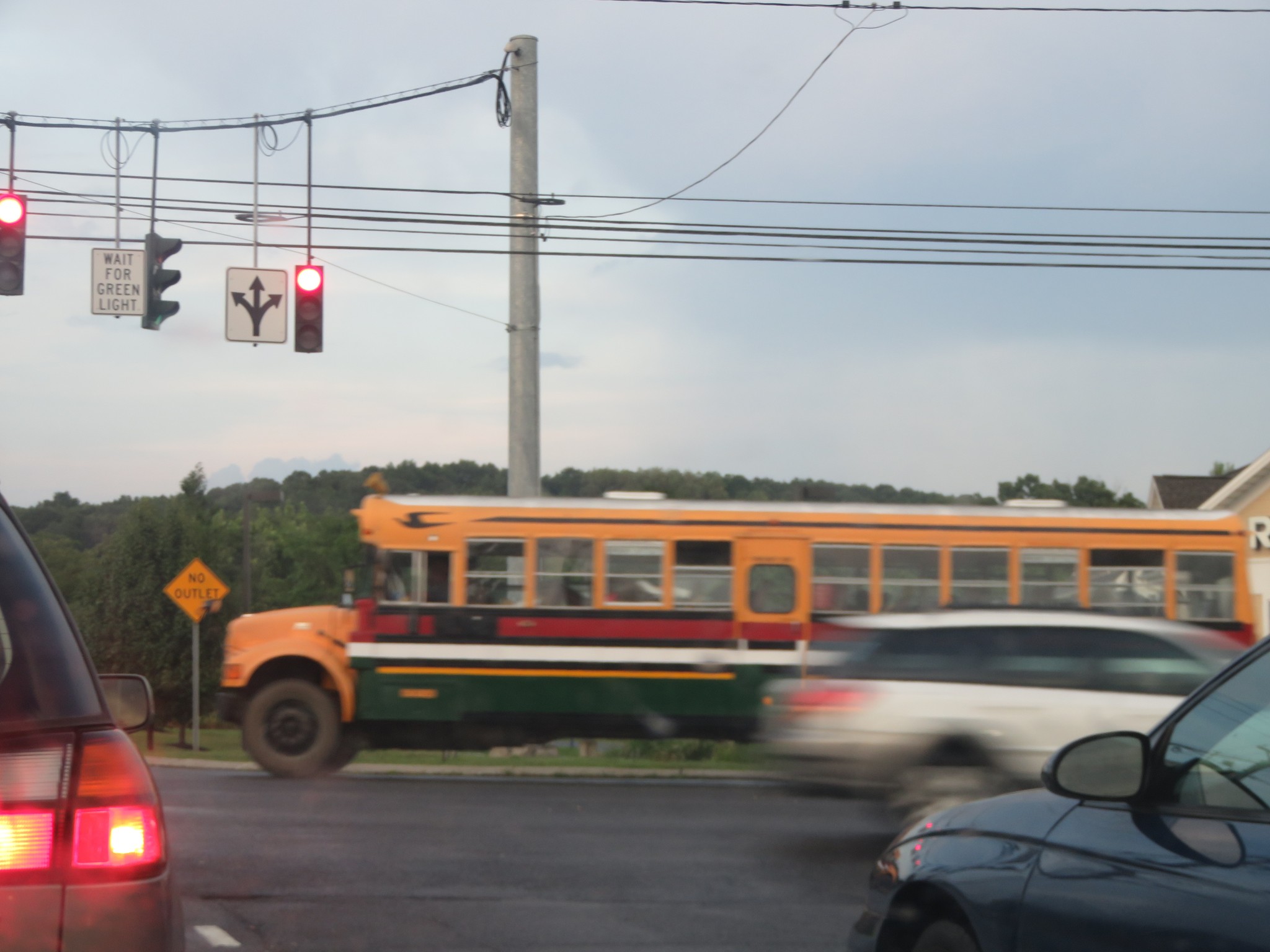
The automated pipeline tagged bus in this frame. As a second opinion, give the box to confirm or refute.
[216,470,1261,779]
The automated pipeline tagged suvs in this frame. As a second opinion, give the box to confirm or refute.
[0,488,183,952]
[759,601,1249,829]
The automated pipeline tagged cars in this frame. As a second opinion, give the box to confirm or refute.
[845,631,1270,952]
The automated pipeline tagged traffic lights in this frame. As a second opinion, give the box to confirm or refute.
[0,193,27,296]
[140,231,181,332]
[294,264,323,353]
